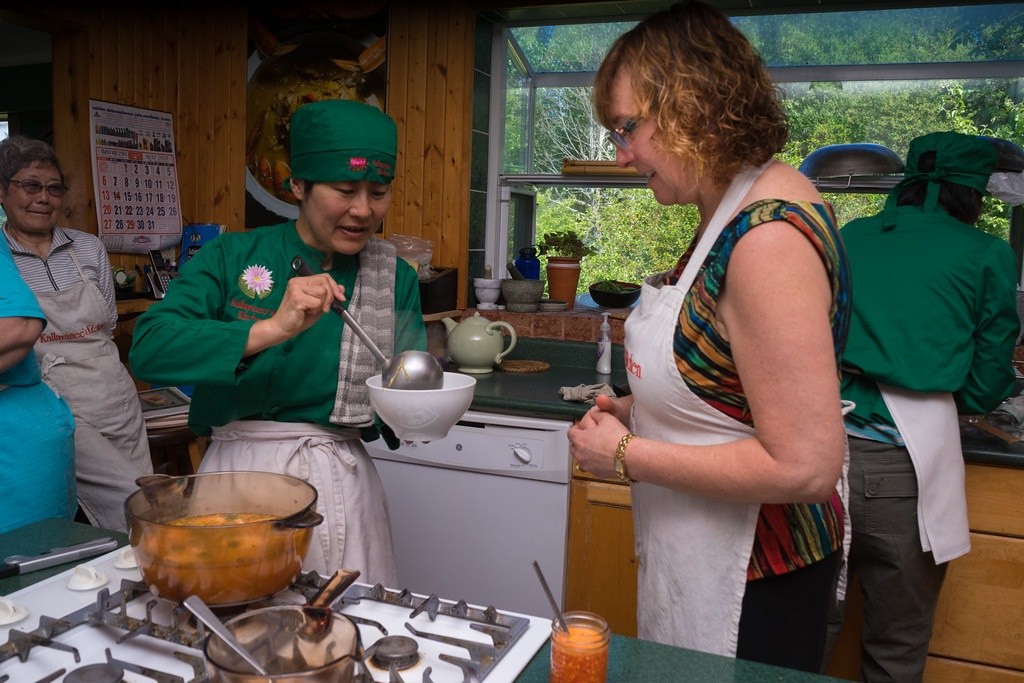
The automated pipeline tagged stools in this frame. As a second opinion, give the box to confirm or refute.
[146,425,209,472]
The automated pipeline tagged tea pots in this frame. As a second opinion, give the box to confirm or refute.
[441,312,516,373]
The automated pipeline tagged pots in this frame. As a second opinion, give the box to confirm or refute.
[124,470,324,607]
[798,143,904,188]
[201,569,365,683]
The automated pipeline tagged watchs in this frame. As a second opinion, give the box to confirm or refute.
[616,433,641,486]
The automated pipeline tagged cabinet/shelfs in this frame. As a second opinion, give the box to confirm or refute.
[564,454,637,639]
[825,463,1024,683]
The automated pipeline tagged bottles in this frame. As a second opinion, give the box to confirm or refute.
[515,244,543,279]
[550,610,611,683]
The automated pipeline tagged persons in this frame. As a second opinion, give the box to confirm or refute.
[567,10,855,672]
[131,99,427,589]
[1,136,153,534]
[0,230,77,535]
[821,130,1021,683]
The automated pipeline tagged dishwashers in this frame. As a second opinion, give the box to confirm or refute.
[359,411,572,619]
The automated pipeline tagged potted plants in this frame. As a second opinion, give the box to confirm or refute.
[539,230,598,308]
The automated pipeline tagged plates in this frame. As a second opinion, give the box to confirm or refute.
[576,291,640,313]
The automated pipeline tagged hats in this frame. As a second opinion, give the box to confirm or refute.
[278,102,399,191]
[880,132,997,230]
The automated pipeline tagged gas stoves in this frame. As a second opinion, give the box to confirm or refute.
[0,542,553,683]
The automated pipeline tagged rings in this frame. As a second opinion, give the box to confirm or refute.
[576,464,584,472]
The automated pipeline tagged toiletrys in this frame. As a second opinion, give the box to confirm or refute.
[594,312,612,375]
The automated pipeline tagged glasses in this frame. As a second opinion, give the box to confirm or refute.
[10,178,65,197]
[605,104,648,145]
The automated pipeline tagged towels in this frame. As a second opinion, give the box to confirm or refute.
[329,234,397,428]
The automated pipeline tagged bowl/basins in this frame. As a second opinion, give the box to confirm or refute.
[365,372,477,442]
[589,281,641,309]
